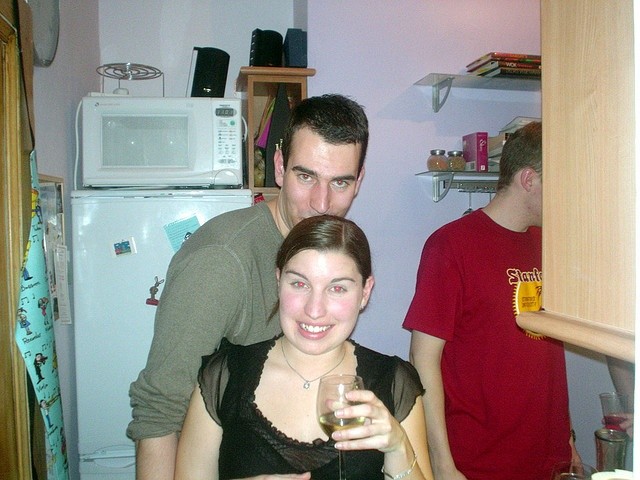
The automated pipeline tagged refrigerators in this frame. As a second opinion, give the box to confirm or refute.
[71,190,254,480]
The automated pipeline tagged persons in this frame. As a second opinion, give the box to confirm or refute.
[174,215,434,480]
[402,122,584,480]
[602,413,633,442]
[124,95,369,479]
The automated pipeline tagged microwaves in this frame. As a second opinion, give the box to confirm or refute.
[81,96,241,189]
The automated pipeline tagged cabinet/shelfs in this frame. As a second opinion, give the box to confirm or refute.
[0,1,35,480]
[514,2,634,362]
[236,68,316,205]
[412,72,541,203]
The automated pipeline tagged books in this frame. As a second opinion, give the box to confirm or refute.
[467,53,542,78]
[486,116,541,174]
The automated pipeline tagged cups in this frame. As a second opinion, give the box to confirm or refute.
[552,463,596,479]
[600,392,629,434]
[594,430,628,471]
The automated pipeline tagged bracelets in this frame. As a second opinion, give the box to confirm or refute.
[381,453,418,479]
[570,429,576,442]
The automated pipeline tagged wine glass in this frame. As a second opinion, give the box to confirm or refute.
[315,374,368,480]
